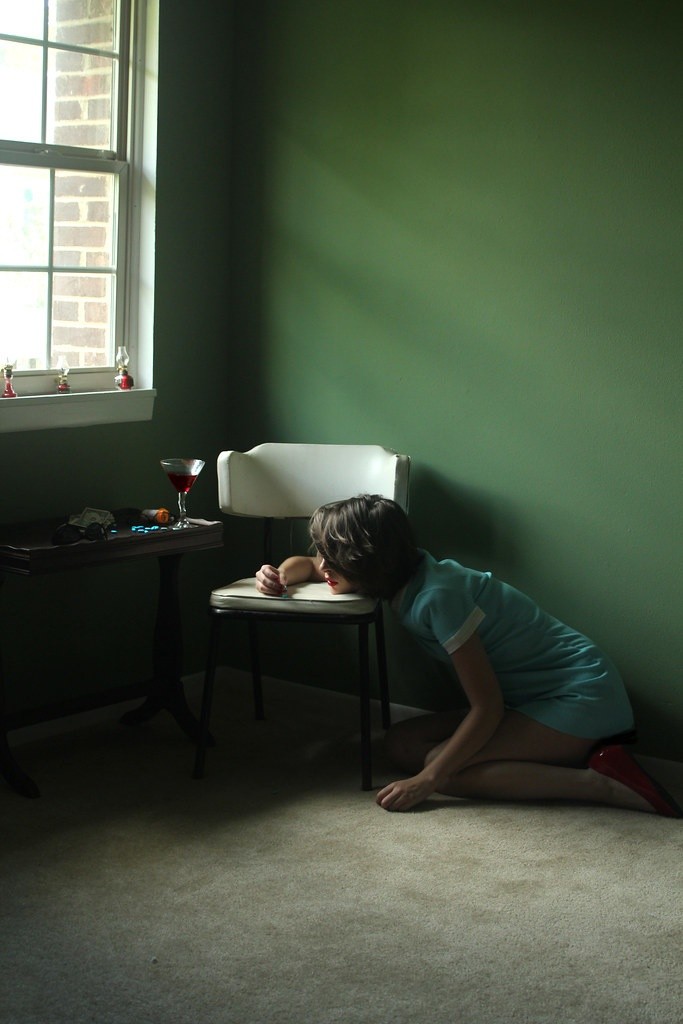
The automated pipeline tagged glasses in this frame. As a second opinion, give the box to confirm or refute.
[129,513,174,534]
[51,523,106,544]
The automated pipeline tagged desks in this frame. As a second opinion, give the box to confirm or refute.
[1,507,216,801]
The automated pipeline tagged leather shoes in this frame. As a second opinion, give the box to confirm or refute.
[588,745,681,817]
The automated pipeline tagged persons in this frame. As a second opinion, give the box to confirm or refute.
[256,495,683,818]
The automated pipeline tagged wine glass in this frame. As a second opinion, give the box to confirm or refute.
[160,458,206,529]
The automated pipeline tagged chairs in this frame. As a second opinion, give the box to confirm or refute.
[192,441,412,792]
